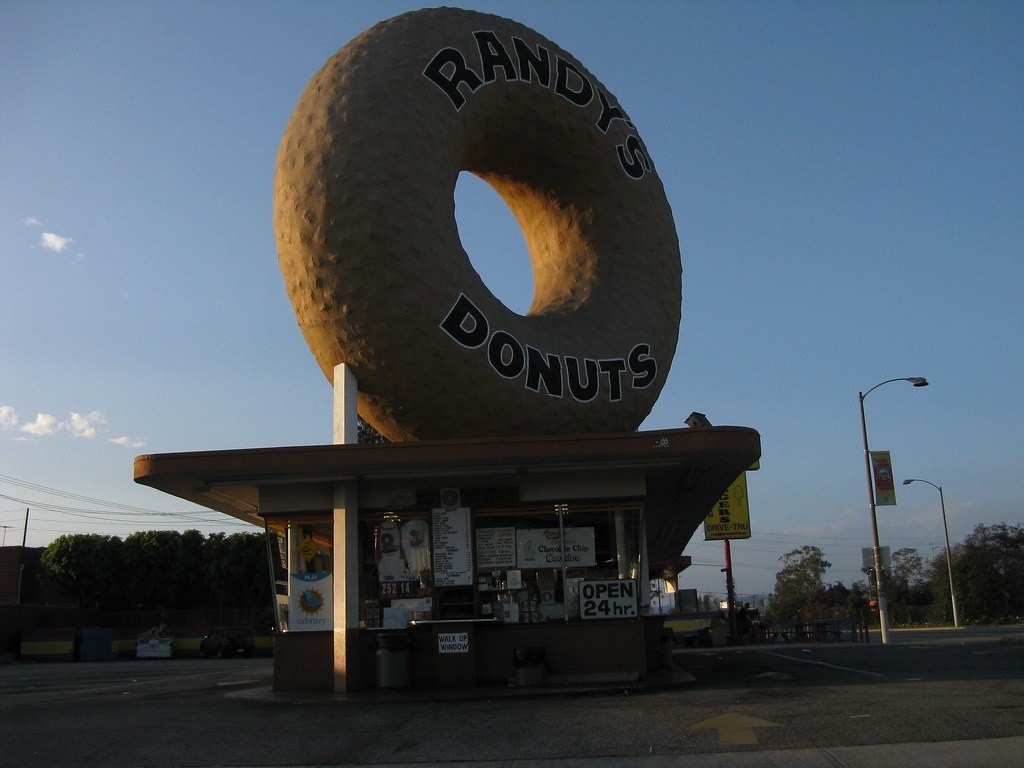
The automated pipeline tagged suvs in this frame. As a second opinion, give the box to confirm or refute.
[199,626,256,660]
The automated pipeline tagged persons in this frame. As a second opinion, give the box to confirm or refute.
[739,603,752,635]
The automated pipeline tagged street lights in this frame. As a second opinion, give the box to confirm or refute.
[857,375,930,643]
[902,478,960,628]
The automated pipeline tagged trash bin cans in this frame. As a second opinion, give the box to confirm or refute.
[513,645,546,687]
[376,633,413,689]
[753,621,767,642]
[80,616,114,661]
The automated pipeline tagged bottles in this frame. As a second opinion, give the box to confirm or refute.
[496,577,501,590]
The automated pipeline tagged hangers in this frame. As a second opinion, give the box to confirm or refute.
[383,519,394,525]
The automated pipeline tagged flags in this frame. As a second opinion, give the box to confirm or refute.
[871,450,897,506]
